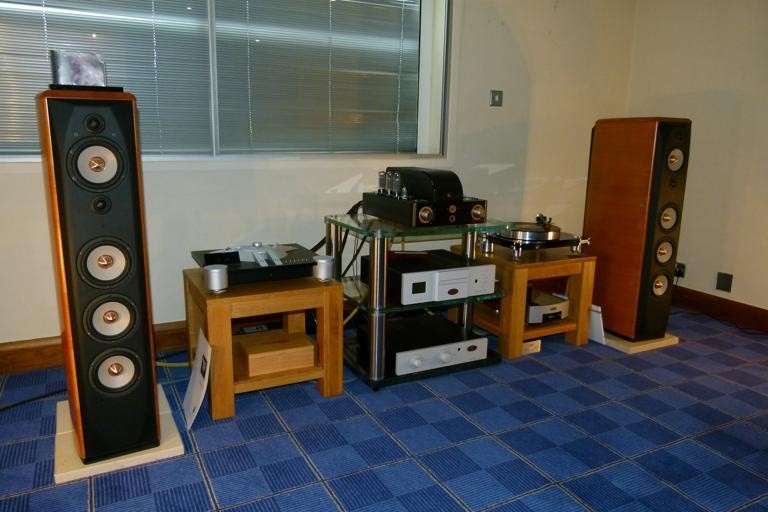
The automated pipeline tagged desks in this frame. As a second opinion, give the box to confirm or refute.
[182,266,345,421]
[450,244,598,360]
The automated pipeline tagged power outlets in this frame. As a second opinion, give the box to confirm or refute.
[674,262,685,277]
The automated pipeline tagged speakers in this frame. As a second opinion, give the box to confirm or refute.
[580,116,692,342]
[35,82,162,465]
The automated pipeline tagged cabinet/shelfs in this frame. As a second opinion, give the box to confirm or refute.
[323,212,513,393]
